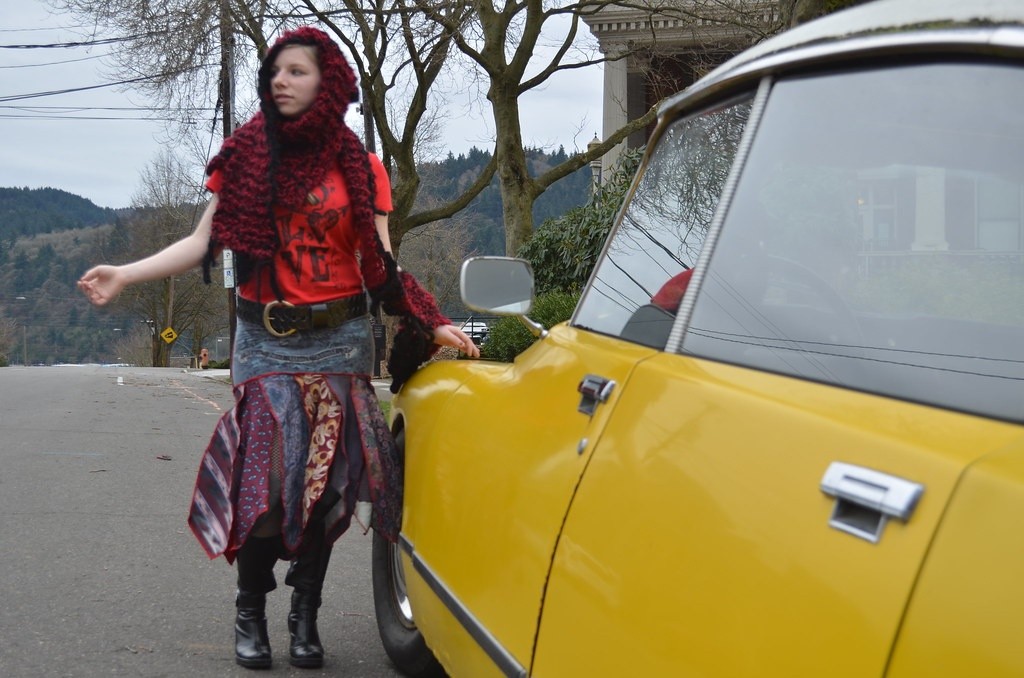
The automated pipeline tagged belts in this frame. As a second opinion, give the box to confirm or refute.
[236,290,371,337]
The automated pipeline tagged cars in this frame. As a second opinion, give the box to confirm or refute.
[370,1,1024,678]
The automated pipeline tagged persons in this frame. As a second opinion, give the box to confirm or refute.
[78,26,481,668]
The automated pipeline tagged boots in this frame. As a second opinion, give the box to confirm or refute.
[233,534,283,669]
[285,520,334,668]
